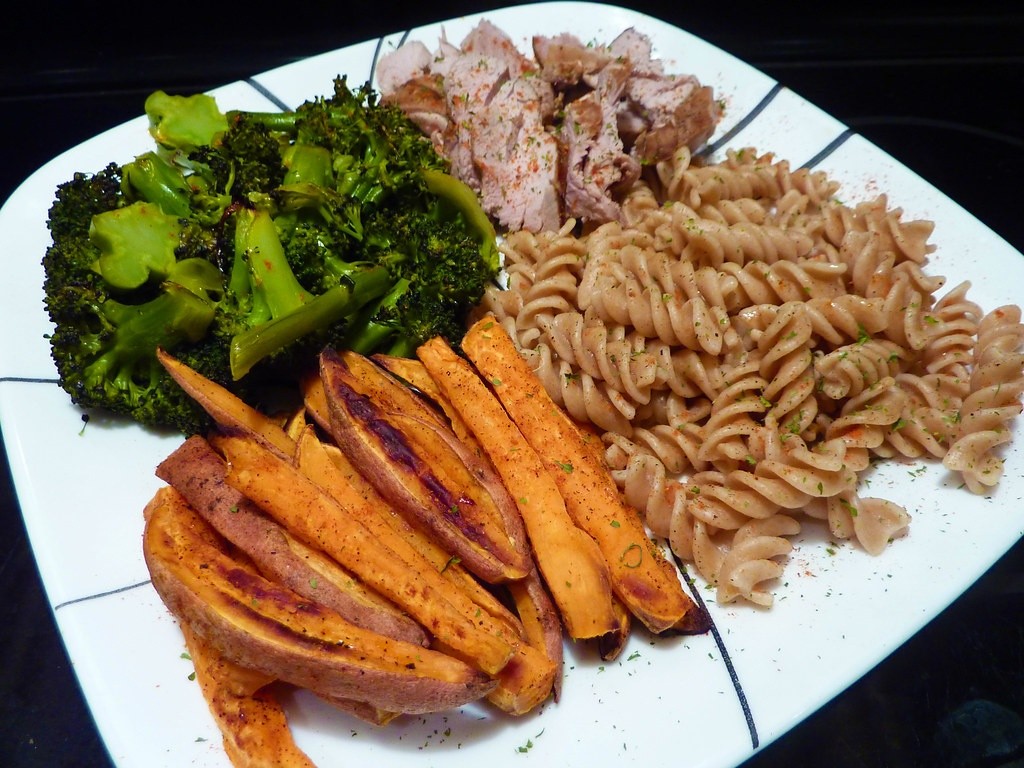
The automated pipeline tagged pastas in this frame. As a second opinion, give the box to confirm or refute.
[485,151,1024,603]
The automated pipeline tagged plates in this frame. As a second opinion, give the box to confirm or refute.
[1,0,1024,768]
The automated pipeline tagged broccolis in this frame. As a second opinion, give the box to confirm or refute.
[45,74,503,431]
